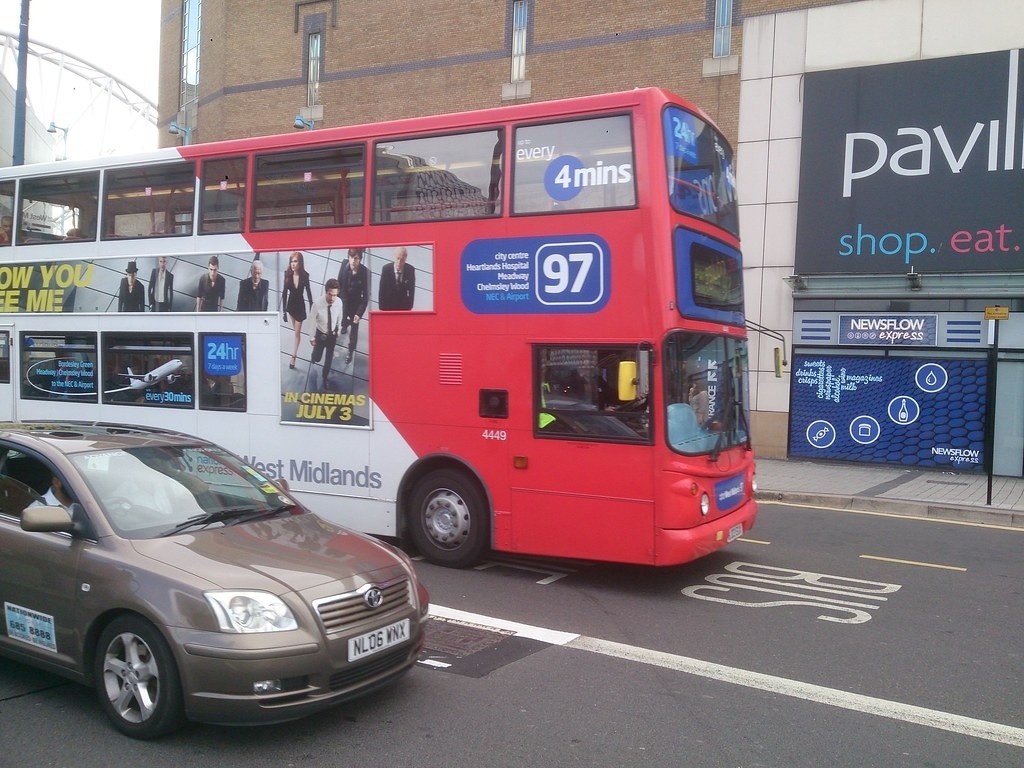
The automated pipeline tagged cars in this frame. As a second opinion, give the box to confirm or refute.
[0,422,429,741]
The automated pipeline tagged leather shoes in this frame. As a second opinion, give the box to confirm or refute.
[345,350,353,364]
[341,326,347,334]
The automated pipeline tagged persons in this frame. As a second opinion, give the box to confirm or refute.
[148,256,174,312]
[27,465,76,517]
[308,279,343,388]
[561,350,648,417]
[118,261,145,312]
[0,216,27,244]
[379,247,415,311]
[237,260,269,312]
[197,256,225,312]
[690,380,709,430]
[338,248,370,363]
[282,252,313,368]
[129,454,209,514]
[66,229,81,240]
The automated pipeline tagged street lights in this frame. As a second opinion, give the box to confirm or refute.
[46,122,69,161]
[168,121,190,146]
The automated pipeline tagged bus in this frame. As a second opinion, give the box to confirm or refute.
[0,86,788,570]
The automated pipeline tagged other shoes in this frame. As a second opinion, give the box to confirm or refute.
[322,377,331,389]
[311,352,318,364]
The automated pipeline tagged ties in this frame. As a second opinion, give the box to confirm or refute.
[397,269,401,282]
[327,305,332,334]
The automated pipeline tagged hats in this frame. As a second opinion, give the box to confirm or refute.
[67,228,89,238]
[125,262,138,273]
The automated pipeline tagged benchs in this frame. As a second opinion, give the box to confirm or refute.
[21,231,81,243]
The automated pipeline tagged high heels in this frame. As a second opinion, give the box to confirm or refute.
[289,355,297,369]
